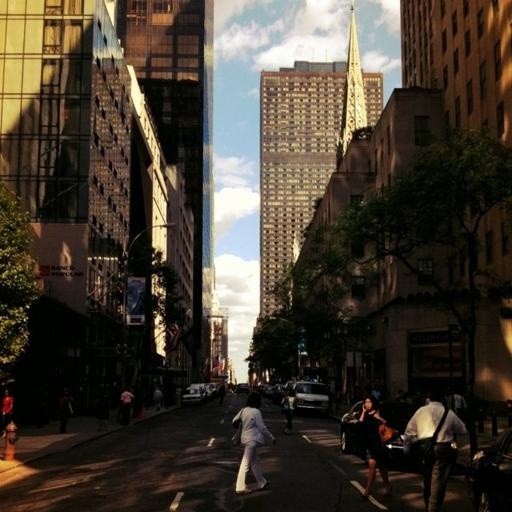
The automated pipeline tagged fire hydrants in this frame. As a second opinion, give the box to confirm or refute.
[3,420,18,461]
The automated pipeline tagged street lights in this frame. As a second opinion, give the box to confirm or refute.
[117,220,177,426]
[269,316,297,379]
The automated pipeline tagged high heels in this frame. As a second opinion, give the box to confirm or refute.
[361,485,392,500]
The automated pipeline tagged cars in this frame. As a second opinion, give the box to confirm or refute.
[462,425,512,512]
[181,380,330,417]
[338,397,427,472]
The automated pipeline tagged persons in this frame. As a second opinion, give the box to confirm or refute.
[60,392,74,434]
[218,385,225,403]
[94,384,164,432]
[230,393,276,493]
[0,388,16,430]
[281,389,297,435]
[357,377,469,512]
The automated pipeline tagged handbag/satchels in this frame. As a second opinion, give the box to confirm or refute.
[377,424,397,444]
[415,453,430,467]
[280,407,289,416]
[231,422,242,447]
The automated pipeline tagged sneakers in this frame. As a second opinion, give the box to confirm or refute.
[235,481,271,494]
